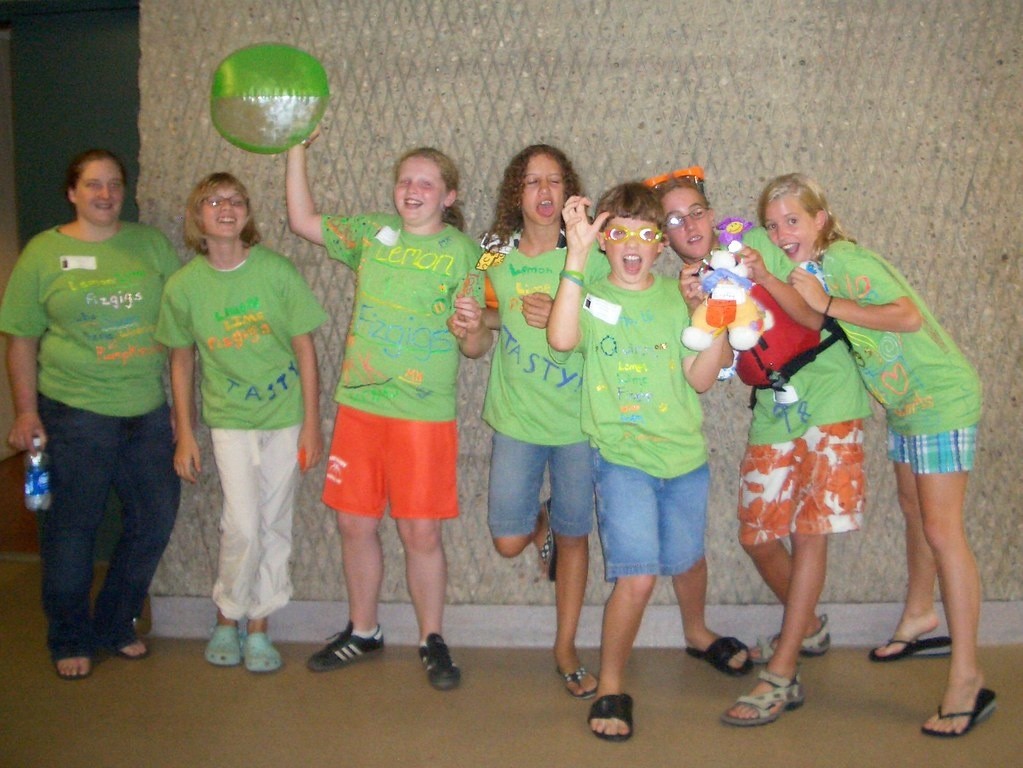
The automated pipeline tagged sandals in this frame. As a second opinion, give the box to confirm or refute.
[239,632,282,673]
[105,636,148,658]
[748,613,830,663]
[53,650,93,678]
[203,625,242,666]
[721,670,804,726]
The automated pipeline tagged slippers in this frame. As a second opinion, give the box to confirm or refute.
[921,689,998,737]
[587,694,633,741]
[869,636,952,661]
[540,498,556,582]
[557,665,599,699]
[686,637,753,675]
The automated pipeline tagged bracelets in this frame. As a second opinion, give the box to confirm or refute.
[561,270,584,287]
[302,139,309,149]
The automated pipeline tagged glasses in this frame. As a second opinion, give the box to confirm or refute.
[663,207,709,229]
[602,224,662,244]
[204,193,246,208]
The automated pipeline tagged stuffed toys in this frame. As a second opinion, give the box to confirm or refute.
[681,250,774,350]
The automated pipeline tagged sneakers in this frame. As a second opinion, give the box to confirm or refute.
[309,620,384,671]
[419,632,459,689]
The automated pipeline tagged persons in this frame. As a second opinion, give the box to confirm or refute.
[547,183,754,742]
[758,173,997,738]
[285,124,494,690]
[0,149,197,680]
[154,172,328,672]
[643,167,873,726]
[446,144,611,700]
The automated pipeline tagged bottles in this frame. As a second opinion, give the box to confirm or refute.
[23,437,52,512]
